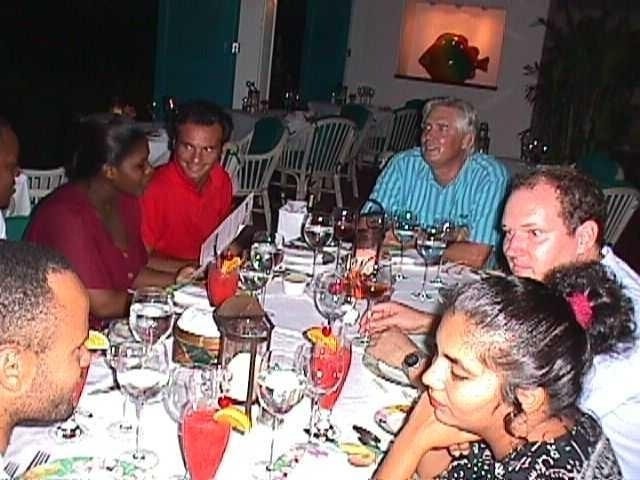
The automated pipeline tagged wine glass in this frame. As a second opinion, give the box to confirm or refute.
[252,337,356,480]
[304,206,450,342]
[203,232,285,318]
[108,287,233,479]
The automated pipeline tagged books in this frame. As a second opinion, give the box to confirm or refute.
[189,191,254,279]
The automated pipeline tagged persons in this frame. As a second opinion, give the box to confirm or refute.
[0,237,93,459]
[356,167,640,479]
[135,96,248,274]
[356,93,509,273]
[18,111,197,319]
[0,112,20,242]
[370,257,638,479]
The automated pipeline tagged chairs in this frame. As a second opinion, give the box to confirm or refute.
[272,104,429,209]
[600,183,639,249]
[0,109,287,249]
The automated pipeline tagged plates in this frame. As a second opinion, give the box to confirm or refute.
[374,405,412,435]
[362,336,436,388]
[280,247,321,266]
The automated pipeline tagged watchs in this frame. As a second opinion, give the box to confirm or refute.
[401,349,431,380]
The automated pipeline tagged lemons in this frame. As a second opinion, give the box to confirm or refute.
[212,407,250,433]
[305,327,337,351]
[85,330,110,350]
[222,256,241,274]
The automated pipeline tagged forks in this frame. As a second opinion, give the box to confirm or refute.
[0,450,51,480]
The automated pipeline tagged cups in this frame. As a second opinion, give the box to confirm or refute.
[49,415,85,440]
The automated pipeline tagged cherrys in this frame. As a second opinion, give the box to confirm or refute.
[218,396,230,408]
[321,325,332,336]
[224,254,233,261]
